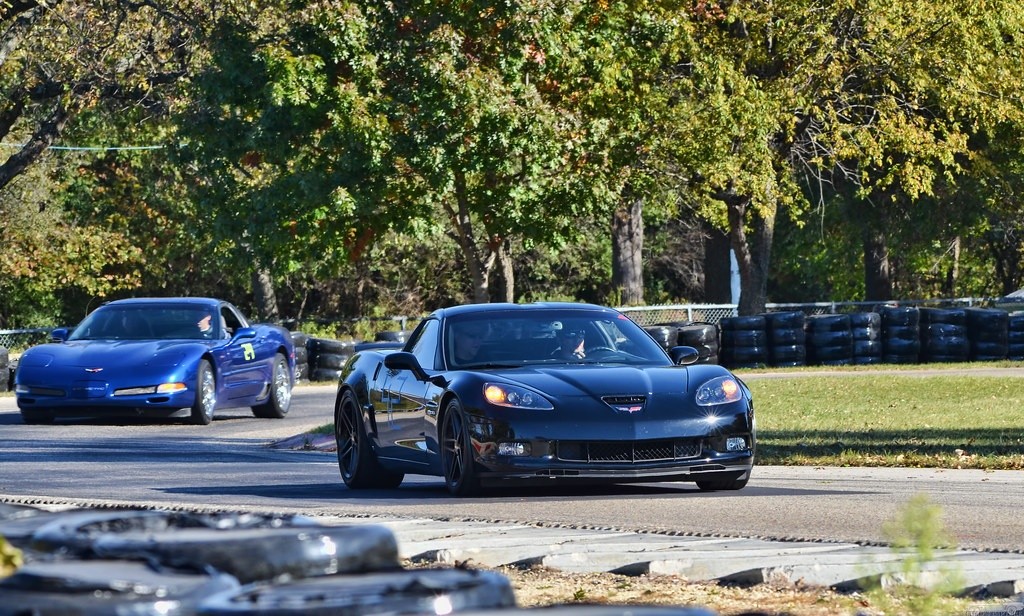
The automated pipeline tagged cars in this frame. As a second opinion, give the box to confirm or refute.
[334,302,762,495]
[14,293,296,425]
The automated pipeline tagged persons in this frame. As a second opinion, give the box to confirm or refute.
[121,310,141,337]
[191,309,225,340]
[455,322,493,364]
[551,321,586,360]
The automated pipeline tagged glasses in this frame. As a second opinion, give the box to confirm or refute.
[461,331,487,339]
[560,329,586,337]
